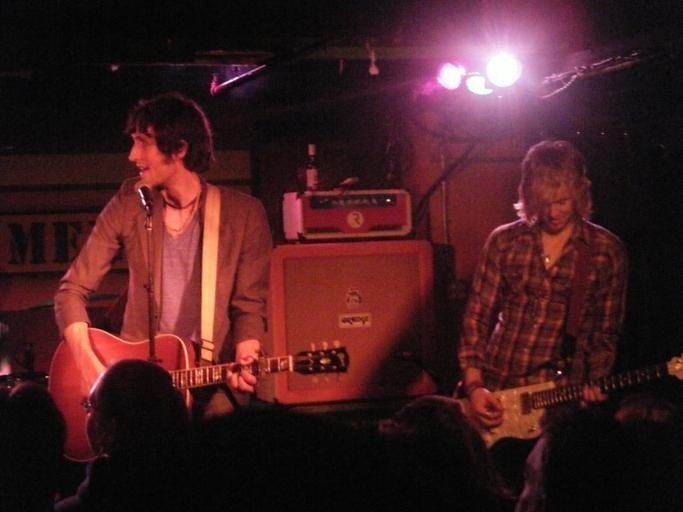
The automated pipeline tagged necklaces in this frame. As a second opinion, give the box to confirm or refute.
[162,193,196,232]
[545,254,551,263]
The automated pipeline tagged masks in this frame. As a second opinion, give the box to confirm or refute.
[85,415,118,456]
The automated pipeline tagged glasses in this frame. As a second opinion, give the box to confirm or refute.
[80,396,100,409]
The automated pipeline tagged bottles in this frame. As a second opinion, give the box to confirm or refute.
[303,144,320,190]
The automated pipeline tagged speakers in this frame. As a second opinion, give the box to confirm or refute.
[256,239,438,406]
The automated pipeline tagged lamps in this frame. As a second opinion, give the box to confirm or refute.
[435,50,523,95]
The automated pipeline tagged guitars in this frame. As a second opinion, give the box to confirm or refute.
[49,325,350,462]
[453,357,682,450]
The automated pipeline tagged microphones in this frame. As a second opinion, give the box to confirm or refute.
[134,179,154,215]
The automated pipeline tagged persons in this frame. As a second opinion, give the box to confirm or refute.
[457,139,629,451]
[55,91,274,415]
[1,360,682,511]
